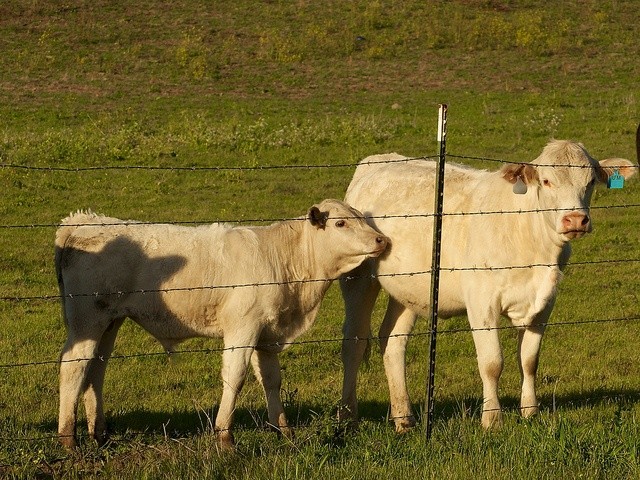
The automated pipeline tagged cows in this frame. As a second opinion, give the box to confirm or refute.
[55,197,390,450]
[339,137,638,434]
